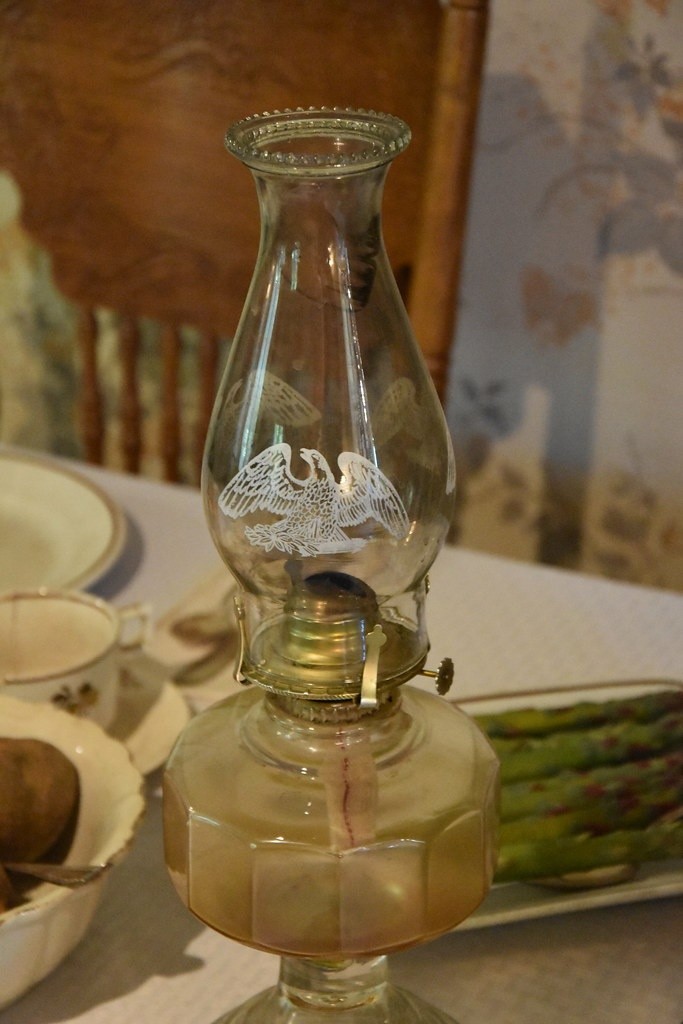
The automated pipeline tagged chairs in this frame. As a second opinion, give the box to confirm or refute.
[0,0,490,515]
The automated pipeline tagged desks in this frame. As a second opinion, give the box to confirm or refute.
[0,447,683,1024]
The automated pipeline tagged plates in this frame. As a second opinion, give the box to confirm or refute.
[105,659,191,776]
[452,677,682,926]
[1,447,127,591]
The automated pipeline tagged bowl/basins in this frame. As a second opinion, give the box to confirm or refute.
[1,695,149,1014]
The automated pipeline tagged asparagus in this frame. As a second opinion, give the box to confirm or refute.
[472,688,683,880]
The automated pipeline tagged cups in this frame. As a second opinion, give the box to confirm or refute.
[0,585,152,729]
